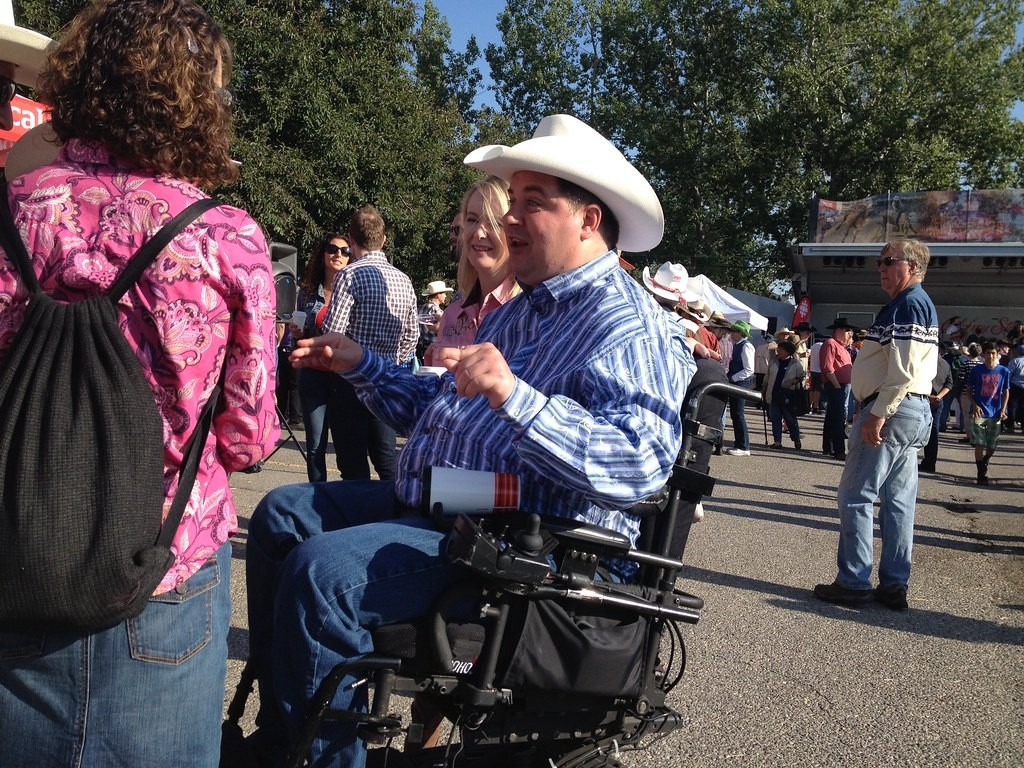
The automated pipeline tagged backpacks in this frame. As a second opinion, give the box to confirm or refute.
[0,197,227,664]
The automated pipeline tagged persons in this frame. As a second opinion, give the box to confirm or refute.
[321,205,419,483]
[424,176,524,369]
[427,209,464,334]
[419,281,456,365]
[0,0,278,767]
[218,113,694,768]
[643,262,1024,484]
[301,235,345,483]
[0,0,58,130]
[816,238,938,610]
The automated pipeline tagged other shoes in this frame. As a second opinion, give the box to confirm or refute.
[918,462,936,473]
[823,449,847,461]
[711,447,722,456]
[982,455,988,476]
[769,441,801,449]
[939,419,1024,443]
[977,476,996,486]
[813,407,824,415]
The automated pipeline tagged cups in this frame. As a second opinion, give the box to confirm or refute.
[292,311,307,332]
[806,349,811,355]
[422,464,522,520]
[416,367,447,376]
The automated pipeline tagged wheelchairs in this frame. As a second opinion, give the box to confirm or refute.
[221,380,765,768]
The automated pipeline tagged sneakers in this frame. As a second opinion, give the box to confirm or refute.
[726,447,751,457]
[814,581,908,614]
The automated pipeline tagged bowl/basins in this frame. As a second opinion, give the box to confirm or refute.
[418,314,437,325]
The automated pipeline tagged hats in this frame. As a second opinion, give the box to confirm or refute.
[761,321,818,355]
[643,261,754,341]
[0,2,68,190]
[825,317,855,330]
[421,280,455,297]
[464,115,665,253]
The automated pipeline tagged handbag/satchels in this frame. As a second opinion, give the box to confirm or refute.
[781,377,812,418]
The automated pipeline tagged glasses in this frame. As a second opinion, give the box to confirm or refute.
[714,327,719,329]
[325,243,352,257]
[730,329,737,333]
[875,256,909,268]
[214,86,232,107]
[777,348,784,351]
[449,226,462,233]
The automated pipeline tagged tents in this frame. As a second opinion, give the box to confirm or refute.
[688,273,769,331]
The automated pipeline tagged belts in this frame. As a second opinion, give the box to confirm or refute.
[860,392,927,410]
